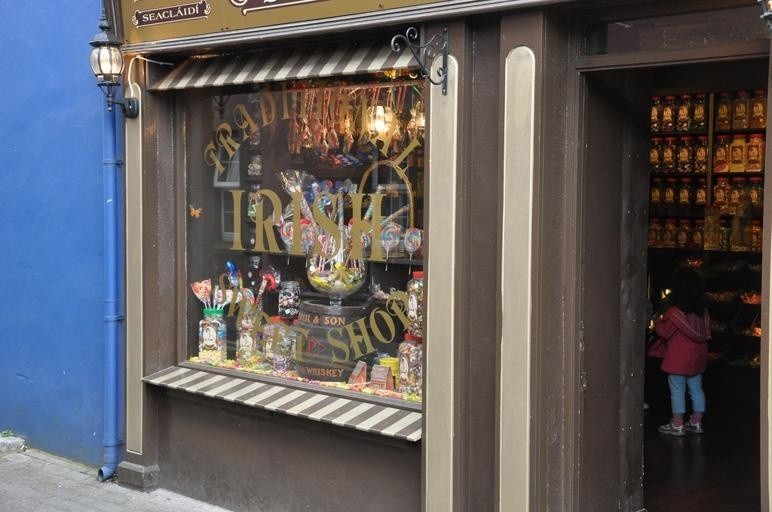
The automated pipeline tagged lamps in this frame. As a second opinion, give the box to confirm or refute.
[406,95,426,142]
[363,88,396,132]
[87,9,141,122]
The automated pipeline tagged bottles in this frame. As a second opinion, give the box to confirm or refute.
[236,311,298,375]
[381,269,426,401]
[648,90,765,250]
[198,307,227,365]
[278,279,300,317]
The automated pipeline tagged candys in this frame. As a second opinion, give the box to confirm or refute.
[398,343,423,395]
[407,279,423,336]
[199,315,293,369]
[190,256,255,308]
[247,161,262,176]
[278,287,301,317]
[248,191,263,216]
[249,126,261,146]
[278,179,423,257]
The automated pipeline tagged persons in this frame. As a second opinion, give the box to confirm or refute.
[650,272,713,437]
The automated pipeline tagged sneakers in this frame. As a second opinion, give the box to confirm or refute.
[685,421,709,435]
[659,422,688,437]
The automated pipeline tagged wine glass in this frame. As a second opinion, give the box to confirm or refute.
[307,235,368,307]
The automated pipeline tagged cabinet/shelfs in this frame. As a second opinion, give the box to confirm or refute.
[220,246,424,311]
[705,86,769,247]
[648,94,714,243]
[645,246,761,373]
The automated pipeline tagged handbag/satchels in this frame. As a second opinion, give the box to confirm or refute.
[648,334,670,364]
